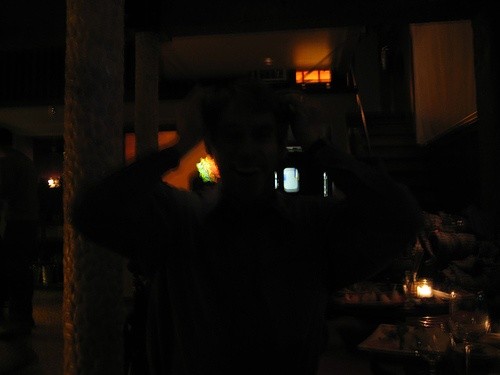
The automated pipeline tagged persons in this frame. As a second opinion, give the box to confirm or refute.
[0,124,40,340]
[74,65,423,375]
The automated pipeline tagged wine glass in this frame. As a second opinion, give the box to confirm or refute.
[446,285,492,374]
[415,314,451,375]
